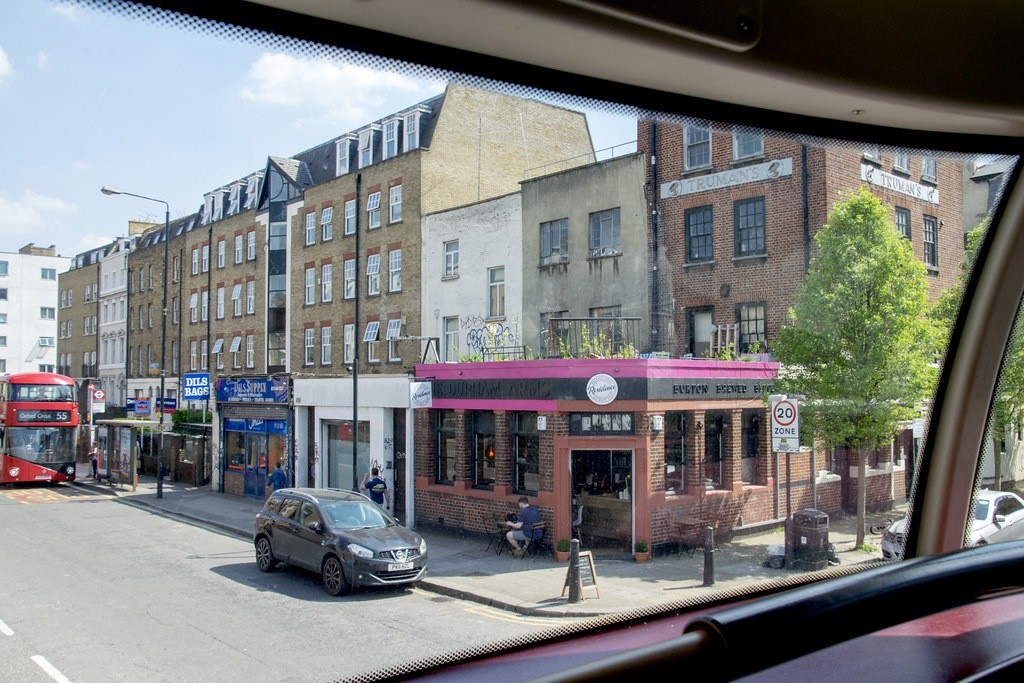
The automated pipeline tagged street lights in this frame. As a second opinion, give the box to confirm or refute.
[101,186,170,498]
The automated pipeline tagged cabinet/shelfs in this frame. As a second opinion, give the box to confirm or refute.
[709,322,739,358]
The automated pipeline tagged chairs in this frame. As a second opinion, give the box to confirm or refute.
[693,518,721,552]
[683,353,693,360]
[521,520,549,562]
[572,505,583,546]
[664,519,690,560]
[481,512,508,556]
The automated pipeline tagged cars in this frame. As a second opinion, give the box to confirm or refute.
[880,488,1024,560]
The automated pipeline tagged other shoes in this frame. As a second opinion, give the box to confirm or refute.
[513,547,527,555]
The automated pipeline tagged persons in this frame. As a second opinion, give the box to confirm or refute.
[88,442,100,481]
[265,462,289,493]
[360,468,390,510]
[505,497,542,556]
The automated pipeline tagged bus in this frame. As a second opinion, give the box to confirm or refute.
[0,372,77,488]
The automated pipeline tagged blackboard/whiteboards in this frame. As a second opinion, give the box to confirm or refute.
[579,551,599,591]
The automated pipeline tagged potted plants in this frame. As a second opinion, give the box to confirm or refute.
[555,539,570,563]
[634,541,649,562]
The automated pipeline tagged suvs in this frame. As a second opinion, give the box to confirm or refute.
[253,486,430,596]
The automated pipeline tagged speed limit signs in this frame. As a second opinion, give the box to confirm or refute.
[774,401,796,426]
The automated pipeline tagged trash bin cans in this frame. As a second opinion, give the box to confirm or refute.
[793,508,830,572]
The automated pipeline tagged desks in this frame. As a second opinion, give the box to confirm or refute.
[675,518,709,558]
[496,520,519,560]
